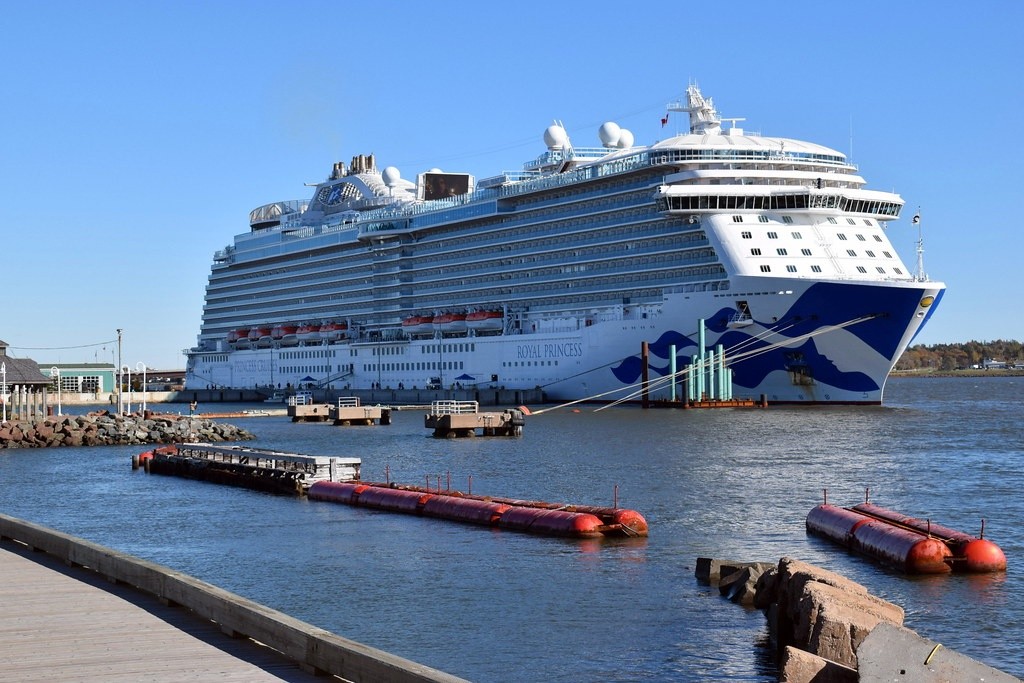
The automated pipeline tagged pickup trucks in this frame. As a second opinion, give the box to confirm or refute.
[140,377,178,391]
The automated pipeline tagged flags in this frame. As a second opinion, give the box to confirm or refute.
[661,112,670,129]
[910,211,919,226]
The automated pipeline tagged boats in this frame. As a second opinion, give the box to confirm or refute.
[402,309,504,335]
[229,321,349,342]
[624,318,772,409]
[264,390,312,404]
[148,442,364,498]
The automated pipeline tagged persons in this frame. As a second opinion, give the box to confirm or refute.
[426,177,456,199]
[398,381,404,391]
[376,382,379,389]
[189,400,198,416]
[278,383,281,389]
[255,383,258,389]
[449,383,455,390]
[206,384,214,390]
[298,383,302,389]
[371,382,375,389]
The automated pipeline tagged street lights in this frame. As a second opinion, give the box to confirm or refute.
[117,328,124,417]
[48,366,63,416]
[0,362,7,424]
[135,361,147,418]
[120,364,132,417]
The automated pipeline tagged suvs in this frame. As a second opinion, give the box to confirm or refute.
[424,376,440,389]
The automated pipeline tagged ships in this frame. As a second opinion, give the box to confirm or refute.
[182,81,947,406]
